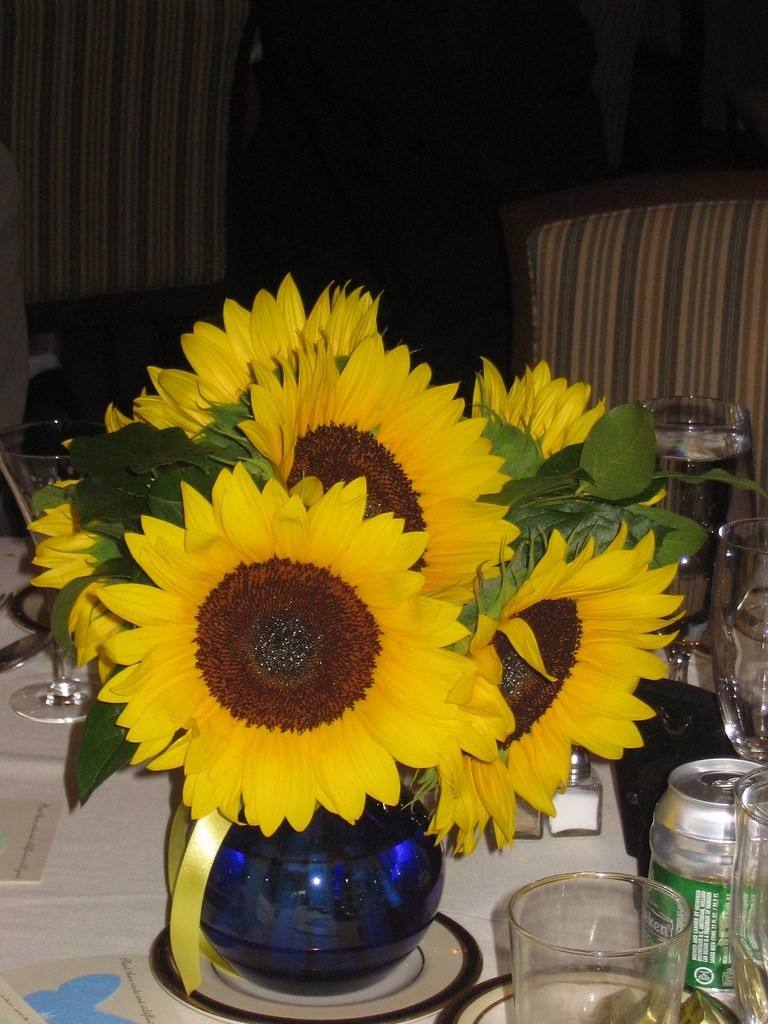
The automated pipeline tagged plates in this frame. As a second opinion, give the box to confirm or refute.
[148,908,485,1024]
[432,964,745,1024]
[9,579,56,636]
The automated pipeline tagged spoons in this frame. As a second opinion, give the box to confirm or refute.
[0,631,53,673]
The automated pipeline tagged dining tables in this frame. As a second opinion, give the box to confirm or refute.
[0,532,731,1024]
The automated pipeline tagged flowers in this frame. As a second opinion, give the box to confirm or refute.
[26,271,768,861]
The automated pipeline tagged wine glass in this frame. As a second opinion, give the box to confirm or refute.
[632,393,757,686]
[0,415,117,726]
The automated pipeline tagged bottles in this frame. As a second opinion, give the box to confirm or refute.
[550,746,603,837]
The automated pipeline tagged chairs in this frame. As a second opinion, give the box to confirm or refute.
[22,275,252,461]
[498,165,768,641]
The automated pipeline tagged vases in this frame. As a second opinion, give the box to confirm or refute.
[201,781,444,998]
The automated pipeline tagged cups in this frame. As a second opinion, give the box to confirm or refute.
[511,871,694,1024]
[732,765,768,1024]
[710,517,768,766]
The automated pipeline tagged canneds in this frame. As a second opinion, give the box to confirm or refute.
[639,756,768,995]
